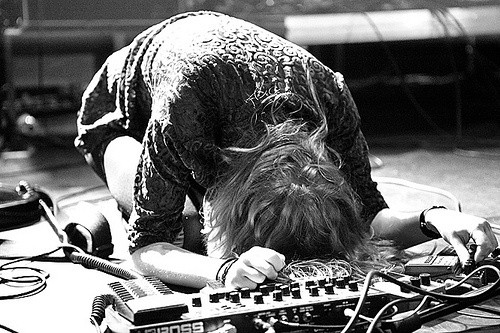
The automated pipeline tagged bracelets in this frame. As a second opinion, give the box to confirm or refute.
[419,205,448,240]
[215,256,238,286]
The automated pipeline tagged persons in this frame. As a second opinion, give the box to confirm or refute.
[74,11,498,290]
